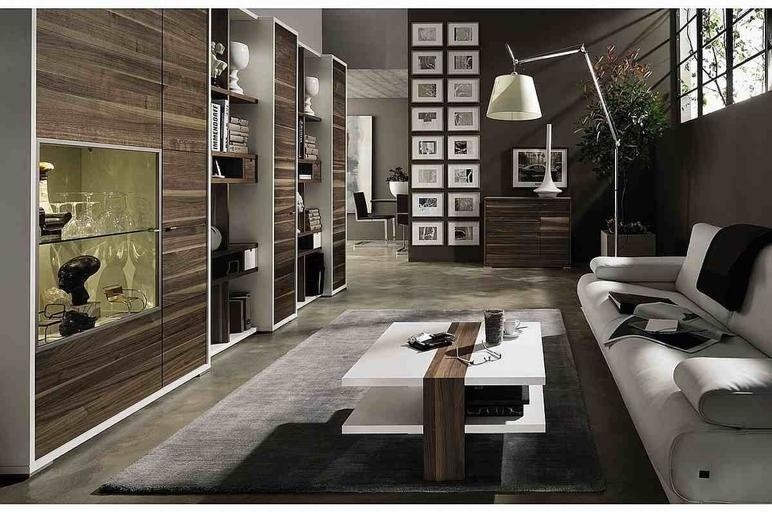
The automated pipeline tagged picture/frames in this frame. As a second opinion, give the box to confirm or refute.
[509,147,569,191]
[410,20,481,249]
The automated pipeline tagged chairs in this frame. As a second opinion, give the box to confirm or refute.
[395,194,409,256]
[353,192,397,248]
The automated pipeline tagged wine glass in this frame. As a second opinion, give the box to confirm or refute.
[230,41,250,92]
[305,75,319,115]
[50,189,146,236]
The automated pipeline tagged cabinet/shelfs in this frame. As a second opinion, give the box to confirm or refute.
[0,8,163,479]
[163,9,208,399]
[293,40,335,319]
[210,8,272,362]
[231,16,298,335]
[306,54,349,300]
[483,194,572,269]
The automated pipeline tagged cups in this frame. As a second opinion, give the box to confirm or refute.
[484,309,504,346]
[503,318,521,334]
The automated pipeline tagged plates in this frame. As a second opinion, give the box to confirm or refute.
[502,334,519,339]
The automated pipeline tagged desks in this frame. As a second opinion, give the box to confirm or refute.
[370,197,410,244]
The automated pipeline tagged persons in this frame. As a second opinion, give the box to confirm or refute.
[298,192,304,213]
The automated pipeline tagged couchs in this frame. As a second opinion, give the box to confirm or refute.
[578,217,771,503]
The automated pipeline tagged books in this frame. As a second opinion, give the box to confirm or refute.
[211,99,249,154]
[464,385,529,416]
[602,301,736,354]
[305,208,322,231]
[305,135,319,161]
[229,292,251,332]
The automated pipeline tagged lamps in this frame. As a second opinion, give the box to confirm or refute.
[483,41,627,264]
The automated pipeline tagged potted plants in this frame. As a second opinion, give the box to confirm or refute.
[571,39,674,256]
[384,165,409,198]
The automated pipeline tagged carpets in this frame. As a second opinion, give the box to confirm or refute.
[88,306,610,499]
